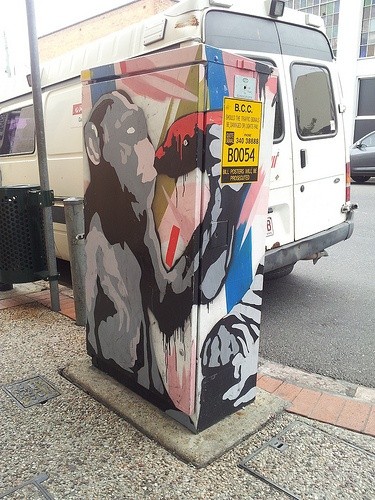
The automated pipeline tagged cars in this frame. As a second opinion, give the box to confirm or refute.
[349,131,375,182]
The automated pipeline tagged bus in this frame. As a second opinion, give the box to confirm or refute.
[0,0,354,295]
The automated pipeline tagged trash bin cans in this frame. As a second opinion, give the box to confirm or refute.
[0,184,62,284]
